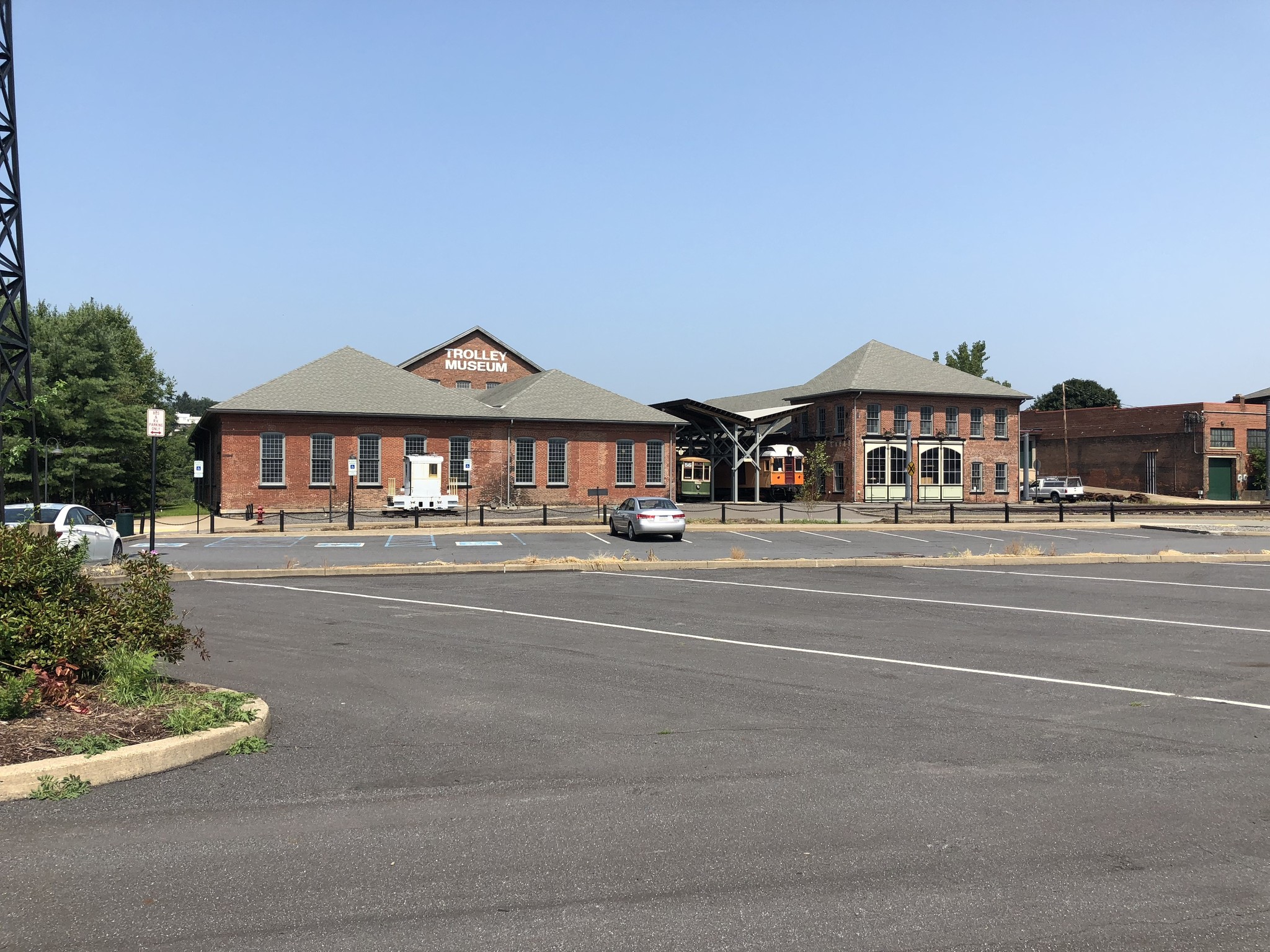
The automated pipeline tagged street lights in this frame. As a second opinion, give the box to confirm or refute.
[72,441,91,504]
[96,443,113,513]
[45,437,64,503]
[113,447,129,518]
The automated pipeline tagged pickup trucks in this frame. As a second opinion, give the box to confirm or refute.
[1020,475,1086,504]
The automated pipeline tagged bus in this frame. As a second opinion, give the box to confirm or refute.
[697,444,805,503]
[676,456,711,500]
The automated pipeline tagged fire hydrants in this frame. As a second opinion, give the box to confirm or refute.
[255,506,266,525]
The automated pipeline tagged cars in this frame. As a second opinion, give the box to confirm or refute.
[4,503,123,566]
[609,497,686,541]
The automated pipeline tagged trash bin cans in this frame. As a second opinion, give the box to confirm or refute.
[115,508,135,537]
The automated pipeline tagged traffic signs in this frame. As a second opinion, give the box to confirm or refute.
[146,409,165,437]
